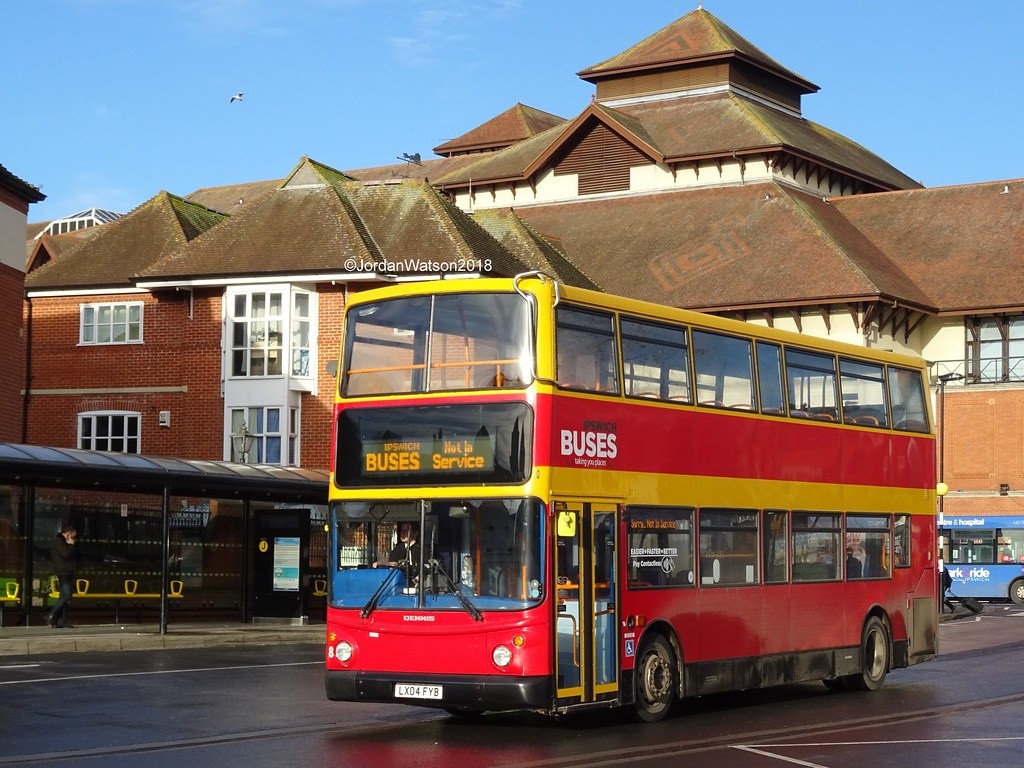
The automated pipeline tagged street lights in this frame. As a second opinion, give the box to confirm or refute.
[936,373,966,614]
[229,419,255,463]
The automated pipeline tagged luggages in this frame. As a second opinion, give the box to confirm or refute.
[947,589,984,614]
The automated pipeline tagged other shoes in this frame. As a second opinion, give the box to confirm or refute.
[950,607,955,614]
[43,611,74,629]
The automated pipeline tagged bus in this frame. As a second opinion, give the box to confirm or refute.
[326,270,942,723]
[936,516,1024,604]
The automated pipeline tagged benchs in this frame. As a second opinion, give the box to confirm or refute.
[46,577,185,598]
[312,580,329,598]
[0,581,26,602]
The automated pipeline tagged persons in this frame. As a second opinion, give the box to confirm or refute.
[370,522,426,591]
[45,520,83,627]
[936,559,956,614]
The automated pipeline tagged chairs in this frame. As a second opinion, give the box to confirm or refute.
[559,381,927,431]
